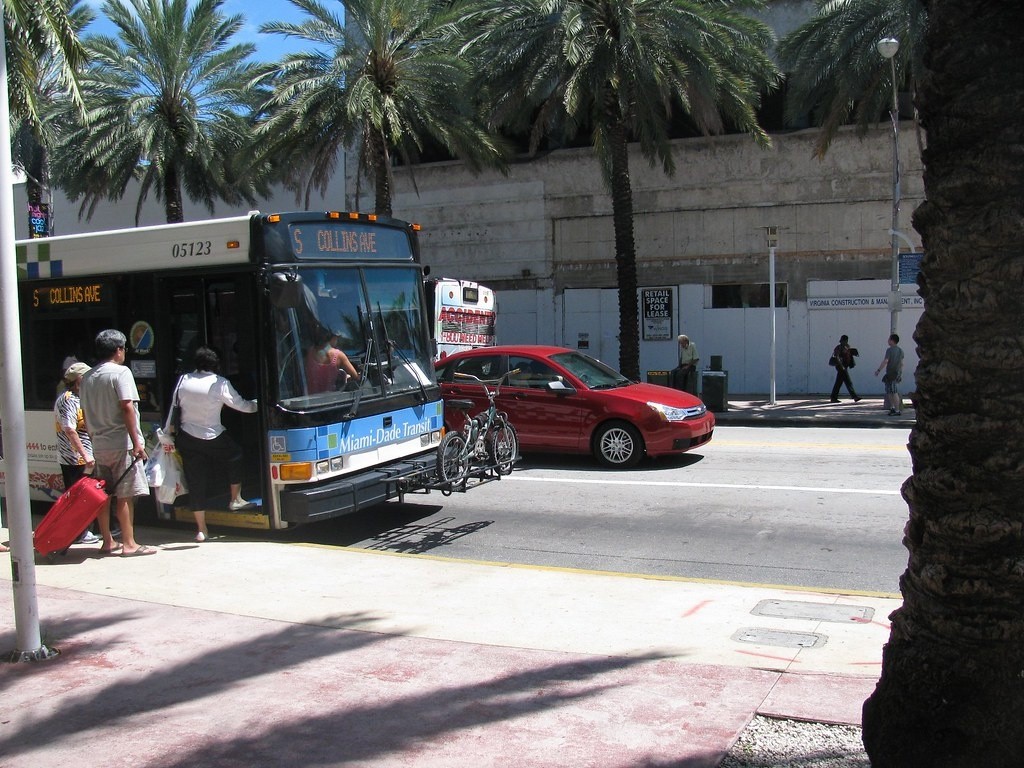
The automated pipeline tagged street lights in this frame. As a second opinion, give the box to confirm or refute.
[877,35,903,409]
[756,224,791,403]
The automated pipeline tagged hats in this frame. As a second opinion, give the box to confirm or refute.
[63,362,92,383]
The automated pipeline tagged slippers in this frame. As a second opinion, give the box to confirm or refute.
[120,545,157,556]
[99,542,123,554]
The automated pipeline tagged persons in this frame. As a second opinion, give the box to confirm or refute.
[830,335,863,403]
[874,333,905,416]
[78,329,160,557]
[54,362,122,544]
[171,345,269,542]
[671,335,699,392]
[305,323,361,394]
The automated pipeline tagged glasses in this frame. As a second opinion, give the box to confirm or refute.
[121,348,128,353]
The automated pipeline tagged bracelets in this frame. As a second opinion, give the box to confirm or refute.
[878,369,880,372]
[354,375,359,381]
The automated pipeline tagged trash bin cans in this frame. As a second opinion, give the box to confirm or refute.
[647,371,671,386]
[702,369,728,414]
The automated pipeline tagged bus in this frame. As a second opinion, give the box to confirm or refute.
[426,277,499,364]
[0,208,523,531]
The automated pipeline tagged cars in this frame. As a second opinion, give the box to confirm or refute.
[434,344,716,472]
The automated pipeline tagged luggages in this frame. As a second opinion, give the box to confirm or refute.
[33,455,142,561]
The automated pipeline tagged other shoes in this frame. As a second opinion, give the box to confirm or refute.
[230,502,257,511]
[855,397,862,402]
[194,532,205,541]
[889,410,901,416]
[831,399,841,403]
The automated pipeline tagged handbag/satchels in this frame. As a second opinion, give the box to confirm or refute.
[829,356,843,366]
[170,406,181,425]
[145,429,188,505]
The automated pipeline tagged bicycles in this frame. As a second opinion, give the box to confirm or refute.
[434,366,521,490]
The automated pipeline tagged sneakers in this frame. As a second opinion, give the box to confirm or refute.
[94,529,120,540]
[75,531,99,543]
